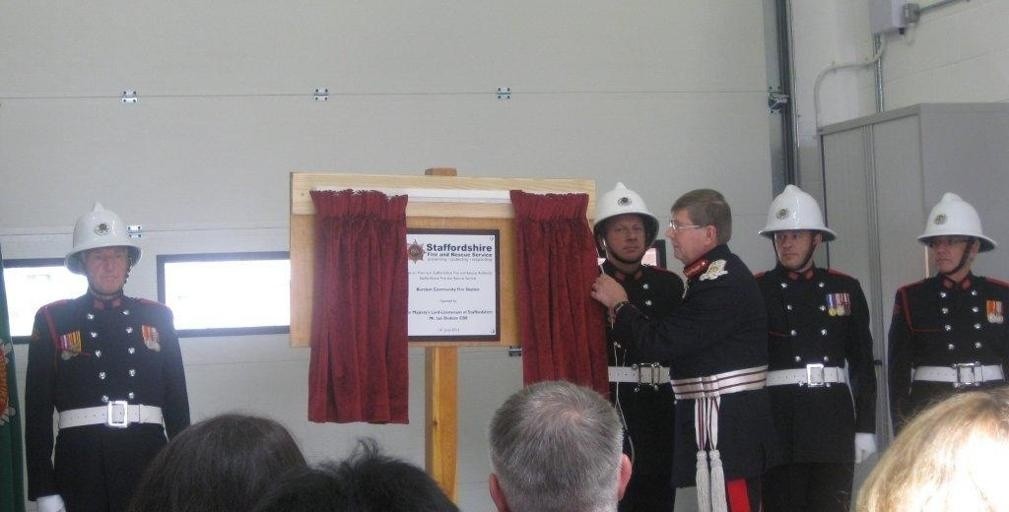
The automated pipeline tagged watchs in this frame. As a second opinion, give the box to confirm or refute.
[612,301,635,314]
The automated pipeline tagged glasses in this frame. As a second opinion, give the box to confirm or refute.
[669,218,701,233]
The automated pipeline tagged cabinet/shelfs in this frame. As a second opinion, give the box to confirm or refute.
[812,102,1007,512]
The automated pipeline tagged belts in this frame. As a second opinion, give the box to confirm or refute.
[766,362,846,388]
[58,399,163,429]
[912,360,1004,387]
[608,362,670,388]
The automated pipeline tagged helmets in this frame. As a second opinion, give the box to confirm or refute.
[758,184,837,242]
[63,201,143,275]
[916,192,998,253]
[589,181,661,259]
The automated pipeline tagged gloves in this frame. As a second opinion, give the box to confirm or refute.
[854,432,879,464]
[36,494,67,512]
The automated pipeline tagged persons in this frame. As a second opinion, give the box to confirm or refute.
[24,201,192,512]
[754,185,876,512]
[594,183,697,511]
[133,415,311,511]
[858,389,1008,511]
[888,191,1009,438]
[250,437,458,512]
[592,183,781,512]
[488,380,632,512]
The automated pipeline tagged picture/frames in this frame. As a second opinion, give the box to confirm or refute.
[404,227,500,341]
